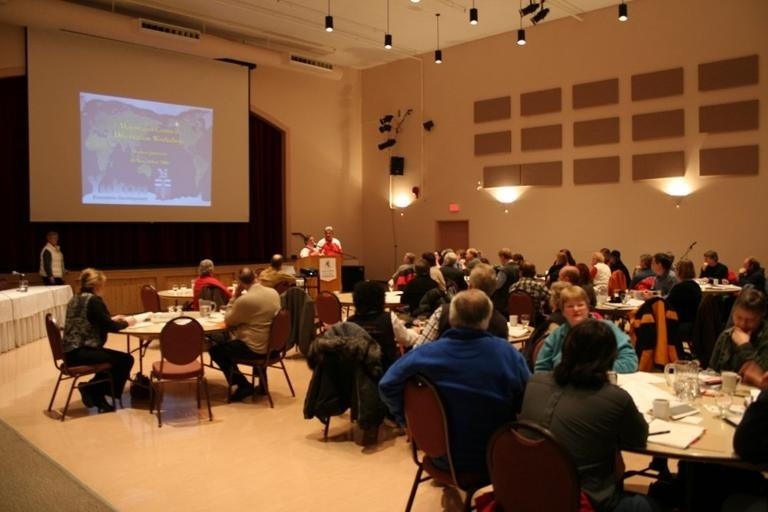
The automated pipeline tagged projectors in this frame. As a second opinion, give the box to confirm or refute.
[299,266,321,276]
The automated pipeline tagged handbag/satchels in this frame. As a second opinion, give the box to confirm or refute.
[130,372,158,403]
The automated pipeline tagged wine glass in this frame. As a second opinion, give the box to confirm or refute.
[715,388,734,421]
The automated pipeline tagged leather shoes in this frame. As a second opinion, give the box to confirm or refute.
[227,383,256,402]
[254,384,266,395]
[94,397,115,412]
[79,381,94,407]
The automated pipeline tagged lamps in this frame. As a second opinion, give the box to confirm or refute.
[616,0,629,23]
[376,114,397,152]
[321,0,337,34]
[381,0,394,51]
[467,1,480,26]
[422,120,434,132]
[519,0,549,27]
[517,0,527,47]
[433,12,443,64]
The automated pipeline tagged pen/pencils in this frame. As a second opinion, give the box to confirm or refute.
[648,430,671,436]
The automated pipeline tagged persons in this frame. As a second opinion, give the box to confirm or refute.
[376,287,533,483]
[532,284,641,375]
[297,235,323,259]
[316,225,344,257]
[257,253,297,290]
[700,388,768,512]
[62,266,135,413]
[37,230,67,288]
[345,280,420,429]
[412,264,510,347]
[210,266,282,405]
[191,259,234,312]
[634,280,708,370]
[705,283,768,392]
[514,319,650,512]
[390,246,768,317]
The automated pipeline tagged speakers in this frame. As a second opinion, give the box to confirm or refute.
[389,156,404,175]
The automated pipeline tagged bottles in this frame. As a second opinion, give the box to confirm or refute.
[18,273,29,292]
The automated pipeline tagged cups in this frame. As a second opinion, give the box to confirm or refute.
[172,282,187,294]
[198,299,217,318]
[652,398,669,421]
[721,372,742,396]
[665,359,699,401]
[509,315,518,327]
[633,290,642,299]
[714,278,718,285]
[520,315,531,329]
